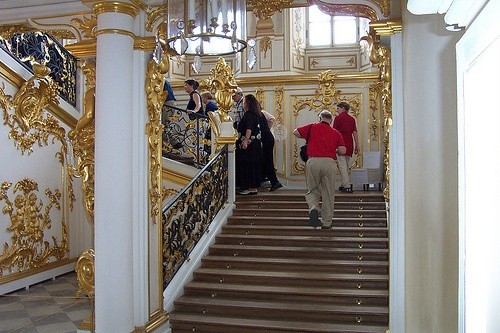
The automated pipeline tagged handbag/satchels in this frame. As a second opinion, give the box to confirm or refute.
[300,123,313,162]
[239,132,264,152]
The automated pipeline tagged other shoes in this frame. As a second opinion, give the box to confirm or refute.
[239,189,258,195]
[270,182,283,192]
[322,224,332,229]
[310,209,318,227]
[339,185,352,192]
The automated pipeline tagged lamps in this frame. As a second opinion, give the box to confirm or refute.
[153,0,257,74]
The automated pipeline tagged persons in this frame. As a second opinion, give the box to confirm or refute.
[259,105,283,193]
[164,81,177,107]
[232,90,245,124]
[331,101,359,193]
[293,110,346,230]
[236,93,265,195]
[201,91,219,115]
[184,79,204,114]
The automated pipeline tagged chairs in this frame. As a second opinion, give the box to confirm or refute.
[350,151,383,192]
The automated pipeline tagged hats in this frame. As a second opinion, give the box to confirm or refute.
[232,87,242,96]
